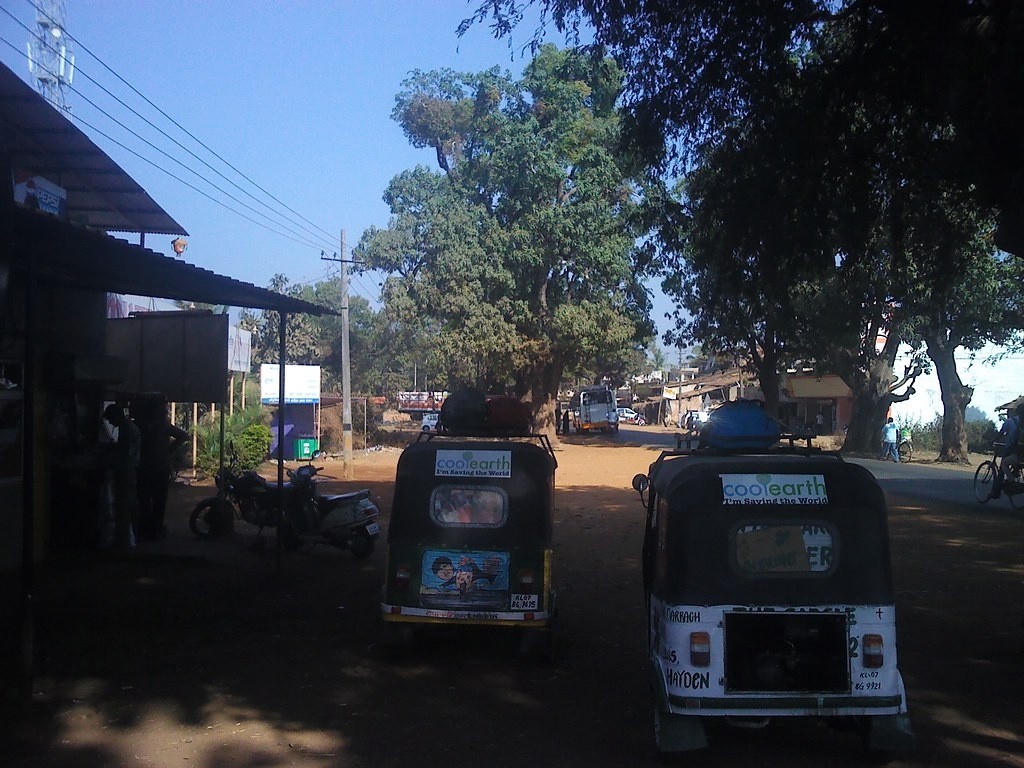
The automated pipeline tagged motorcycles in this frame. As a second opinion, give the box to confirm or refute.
[188,439,329,539]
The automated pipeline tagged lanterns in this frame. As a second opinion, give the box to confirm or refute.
[170,239,188,257]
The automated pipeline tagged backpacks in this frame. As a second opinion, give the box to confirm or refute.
[699,398,781,451]
[441,388,535,435]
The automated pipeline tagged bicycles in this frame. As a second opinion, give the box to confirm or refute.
[974,441,1024,511]
[880,436,913,463]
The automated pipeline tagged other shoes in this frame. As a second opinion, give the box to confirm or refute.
[988,492,1000,499]
[894,461,898,463]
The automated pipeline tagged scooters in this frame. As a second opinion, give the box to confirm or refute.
[276,449,380,558]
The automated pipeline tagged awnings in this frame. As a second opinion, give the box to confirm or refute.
[0,59,341,318]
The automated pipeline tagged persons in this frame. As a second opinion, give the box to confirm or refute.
[880,417,898,463]
[988,403,1024,498]
[103,394,187,550]
[607,409,618,432]
[813,412,824,435]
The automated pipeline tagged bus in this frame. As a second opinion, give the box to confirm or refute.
[569,385,618,434]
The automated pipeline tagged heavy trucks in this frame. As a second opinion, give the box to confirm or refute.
[396,390,452,421]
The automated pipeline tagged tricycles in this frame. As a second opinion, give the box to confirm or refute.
[380,387,558,665]
[633,396,915,765]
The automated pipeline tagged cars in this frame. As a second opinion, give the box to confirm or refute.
[421,414,443,432]
[616,408,649,426]
